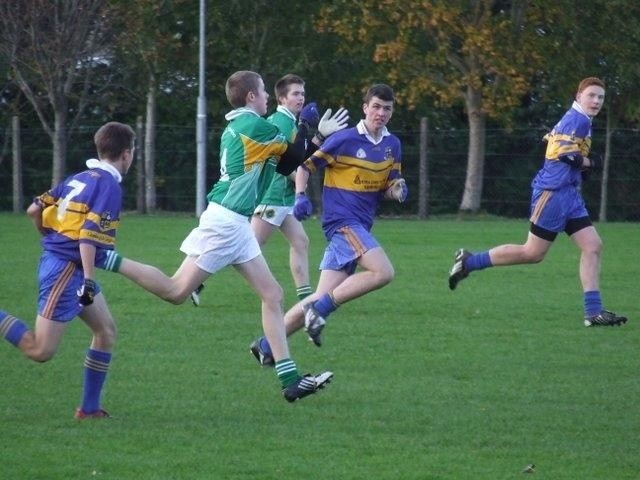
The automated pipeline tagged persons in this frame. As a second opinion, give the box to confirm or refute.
[0,122,136,420]
[250,83,407,366]
[94,71,349,402]
[188,73,311,306]
[449,78,627,328]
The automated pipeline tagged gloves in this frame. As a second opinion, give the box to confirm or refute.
[588,152,603,170]
[315,107,349,142]
[293,192,312,221]
[77,279,96,308]
[300,102,319,127]
[390,178,407,204]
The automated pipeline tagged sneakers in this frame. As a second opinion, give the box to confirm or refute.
[301,301,326,347]
[448,248,471,290]
[584,311,628,328]
[249,338,274,367]
[282,370,334,403]
[74,408,110,420]
[189,283,207,306]
